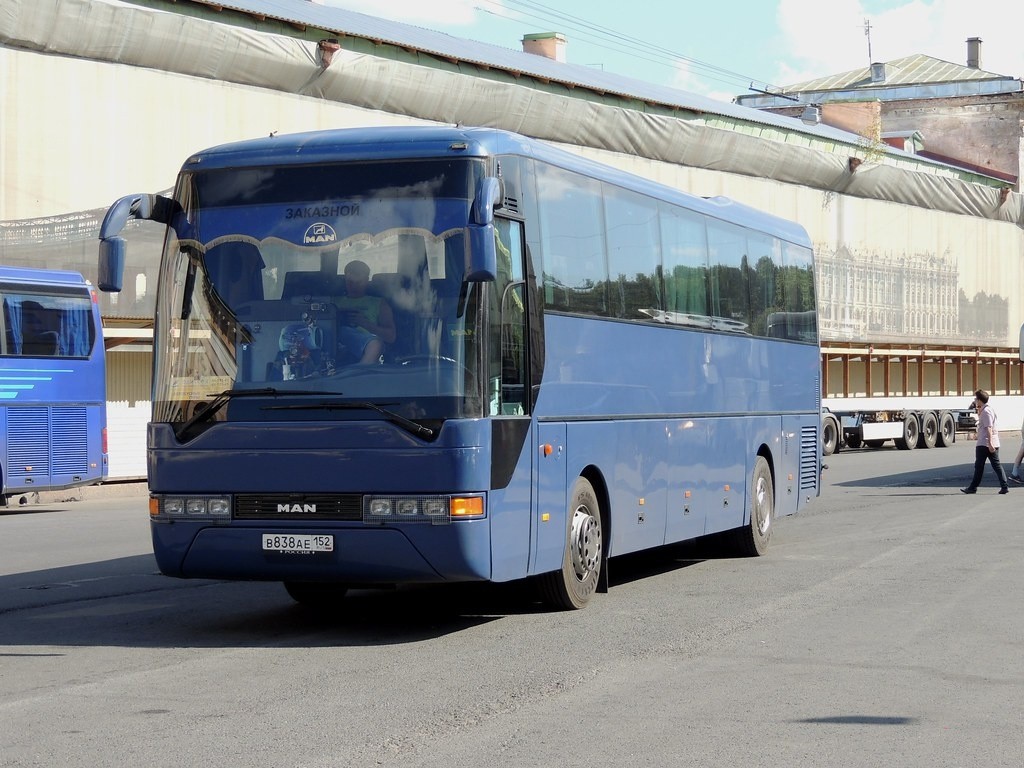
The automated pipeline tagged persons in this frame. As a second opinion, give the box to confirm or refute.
[1008,419,1024,483]
[325,259,396,367]
[959,390,1009,494]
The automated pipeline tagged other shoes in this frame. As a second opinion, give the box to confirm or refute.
[960,487,976,494]
[1008,473,1024,483]
[999,487,1008,494]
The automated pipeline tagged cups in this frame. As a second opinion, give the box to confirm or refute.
[282,365,290,381]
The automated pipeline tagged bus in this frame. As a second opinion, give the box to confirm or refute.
[0,267,110,506]
[97,125,827,609]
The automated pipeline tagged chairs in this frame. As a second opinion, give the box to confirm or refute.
[30,331,60,356]
[372,272,422,368]
[281,270,320,296]
[335,274,372,297]
